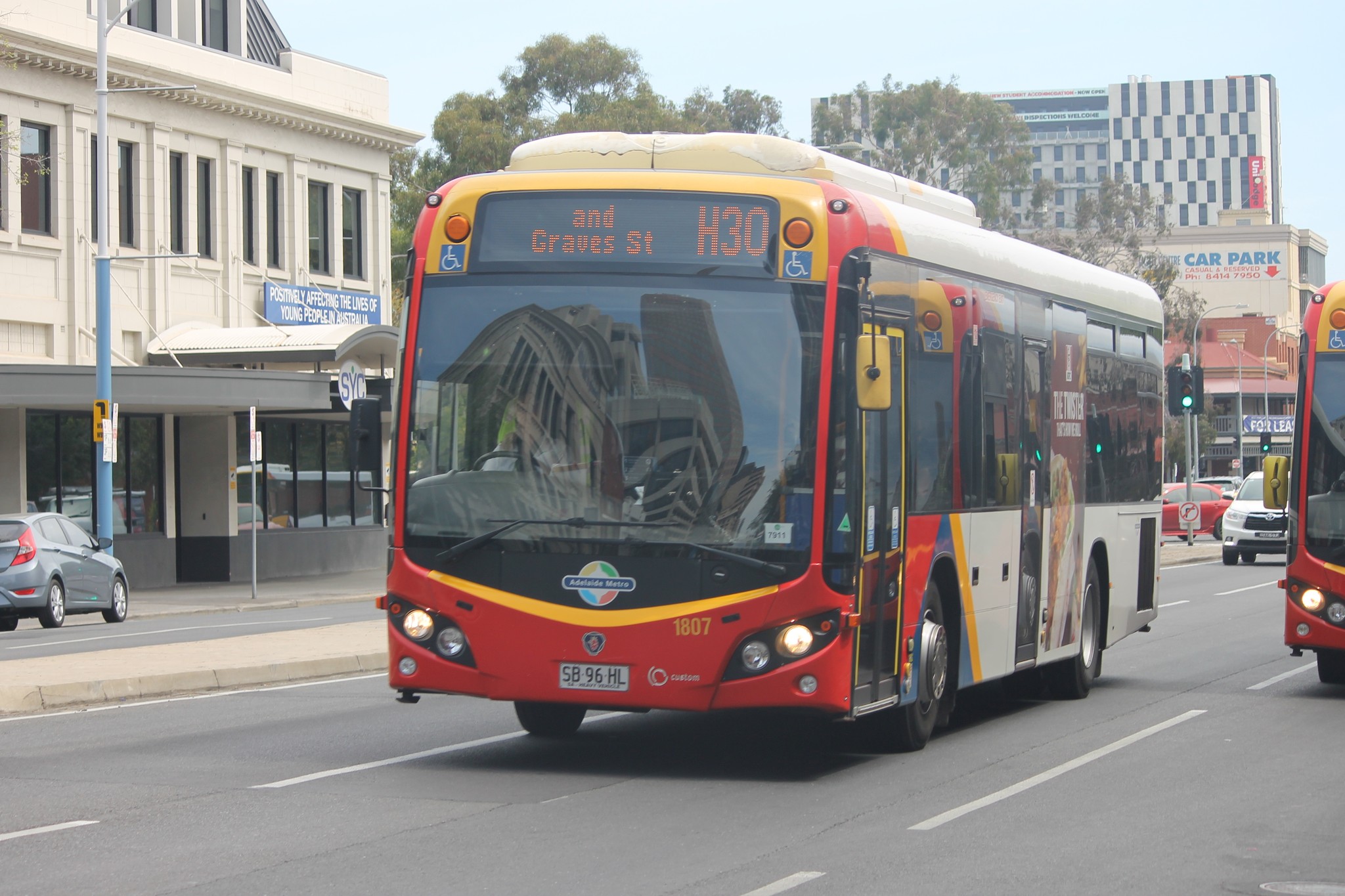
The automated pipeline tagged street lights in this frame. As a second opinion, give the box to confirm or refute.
[1229,338,1245,481]
[1191,303,1250,483]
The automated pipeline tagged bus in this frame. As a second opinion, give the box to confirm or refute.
[237,463,418,529]
[1265,278,1345,683]
[348,134,1163,754]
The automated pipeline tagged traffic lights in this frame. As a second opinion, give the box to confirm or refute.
[1260,433,1272,453]
[1167,366,1204,416]
[1232,434,1241,451]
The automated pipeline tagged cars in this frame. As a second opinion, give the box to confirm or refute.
[236,502,285,529]
[27,483,161,537]
[1219,470,1289,566]
[0,511,130,632]
[1163,475,1244,541]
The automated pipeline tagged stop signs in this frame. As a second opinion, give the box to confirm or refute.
[1232,459,1240,469]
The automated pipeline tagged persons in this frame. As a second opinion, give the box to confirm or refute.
[479,392,580,482]
